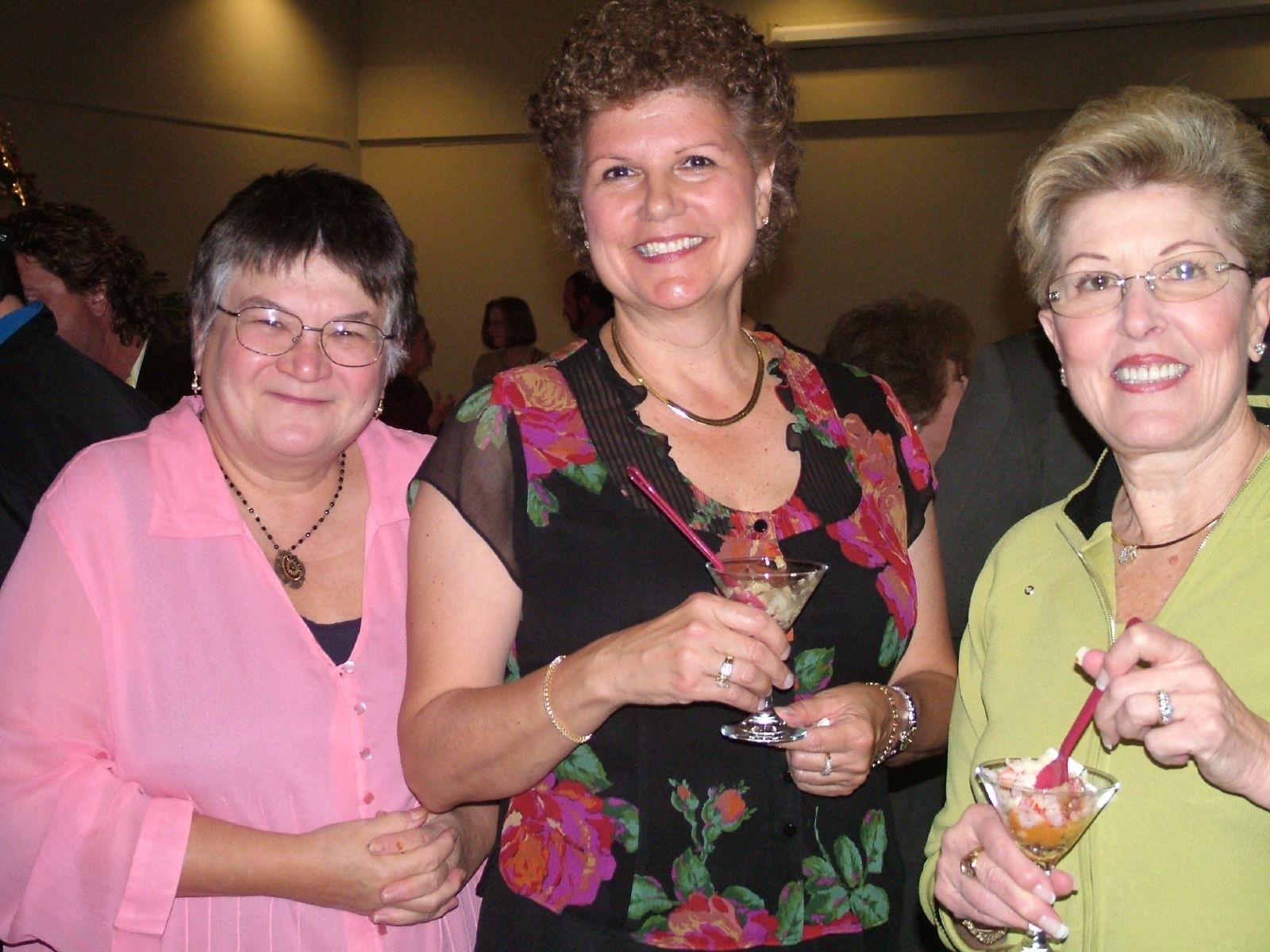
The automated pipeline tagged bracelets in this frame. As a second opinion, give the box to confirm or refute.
[865,682,900,767]
[542,656,591,742]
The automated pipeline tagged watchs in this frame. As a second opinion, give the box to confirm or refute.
[889,685,917,760]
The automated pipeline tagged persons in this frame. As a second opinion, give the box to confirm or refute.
[0,164,504,952]
[199,411,347,589]
[398,0,956,952]
[917,85,1270,952]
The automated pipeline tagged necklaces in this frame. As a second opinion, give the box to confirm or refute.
[0,200,1270,863]
[611,318,764,426]
[1112,513,1222,566]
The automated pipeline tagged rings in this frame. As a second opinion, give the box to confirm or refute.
[715,655,734,688]
[960,847,985,878]
[822,753,833,775]
[1157,690,1172,724]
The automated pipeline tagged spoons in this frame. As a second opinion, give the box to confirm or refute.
[1034,617,1143,790]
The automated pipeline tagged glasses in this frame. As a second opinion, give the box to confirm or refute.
[1046,250,1256,318]
[213,301,397,368]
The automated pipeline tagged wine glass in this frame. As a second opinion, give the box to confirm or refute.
[976,758,1120,952]
[705,557,829,743]
